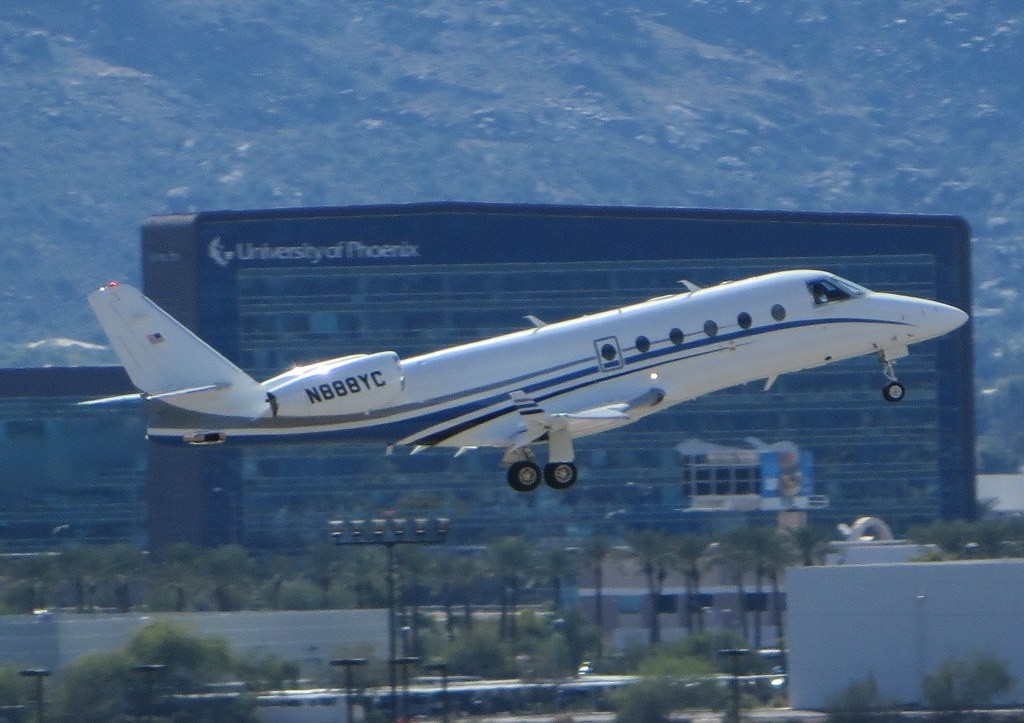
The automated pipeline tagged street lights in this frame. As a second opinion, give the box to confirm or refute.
[694,542,723,633]
[330,516,452,723]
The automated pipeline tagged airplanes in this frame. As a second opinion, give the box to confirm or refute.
[69,269,970,492]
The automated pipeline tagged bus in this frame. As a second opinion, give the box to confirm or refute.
[715,649,787,689]
